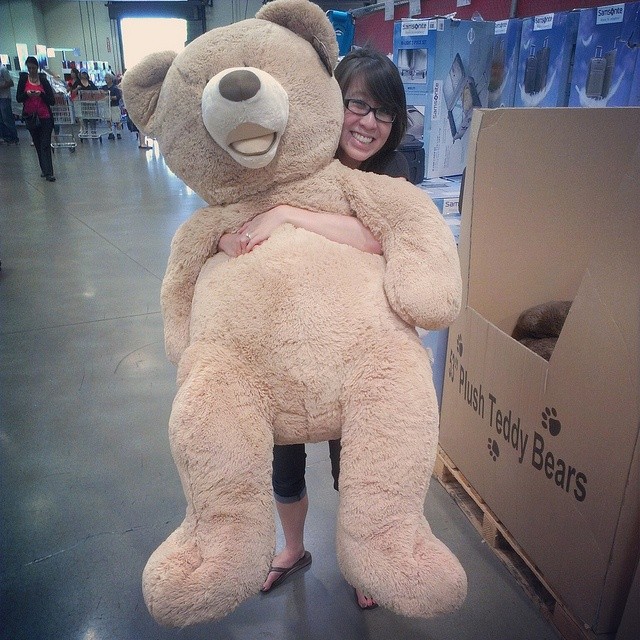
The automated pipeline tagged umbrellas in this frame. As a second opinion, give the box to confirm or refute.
[46,175,56,181]
[40,173,45,177]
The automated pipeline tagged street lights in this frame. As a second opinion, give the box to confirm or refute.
[24,112,41,130]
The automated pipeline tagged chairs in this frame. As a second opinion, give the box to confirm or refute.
[108,133,115,139]
[117,134,121,139]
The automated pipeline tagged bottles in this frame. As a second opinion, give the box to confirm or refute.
[80,68,88,74]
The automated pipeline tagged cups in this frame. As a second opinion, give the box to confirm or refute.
[343,99,400,123]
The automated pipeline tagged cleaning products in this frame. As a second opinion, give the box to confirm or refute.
[47,92,78,155]
[79,89,113,144]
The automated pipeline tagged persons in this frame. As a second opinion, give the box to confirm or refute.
[128,112,153,149]
[260,48,411,609]
[16,56,56,182]
[53,124,60,143]
[71,68,98,134]
[66,68,83,133]
[99,73,123,140]
[0,56,19,146]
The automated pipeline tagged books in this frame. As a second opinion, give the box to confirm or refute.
[416,173,463,218]
[568,0,639,106]
[489,17,521,108]
[392,15,495,180]
[418,326,449,417]
[438,106,640,638]
[514,8,579,107]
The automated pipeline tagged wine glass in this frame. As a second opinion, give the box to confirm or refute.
[244,233,252,239]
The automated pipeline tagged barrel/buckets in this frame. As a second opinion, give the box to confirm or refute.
[354,588,379,610]
[262,550,312,592]
